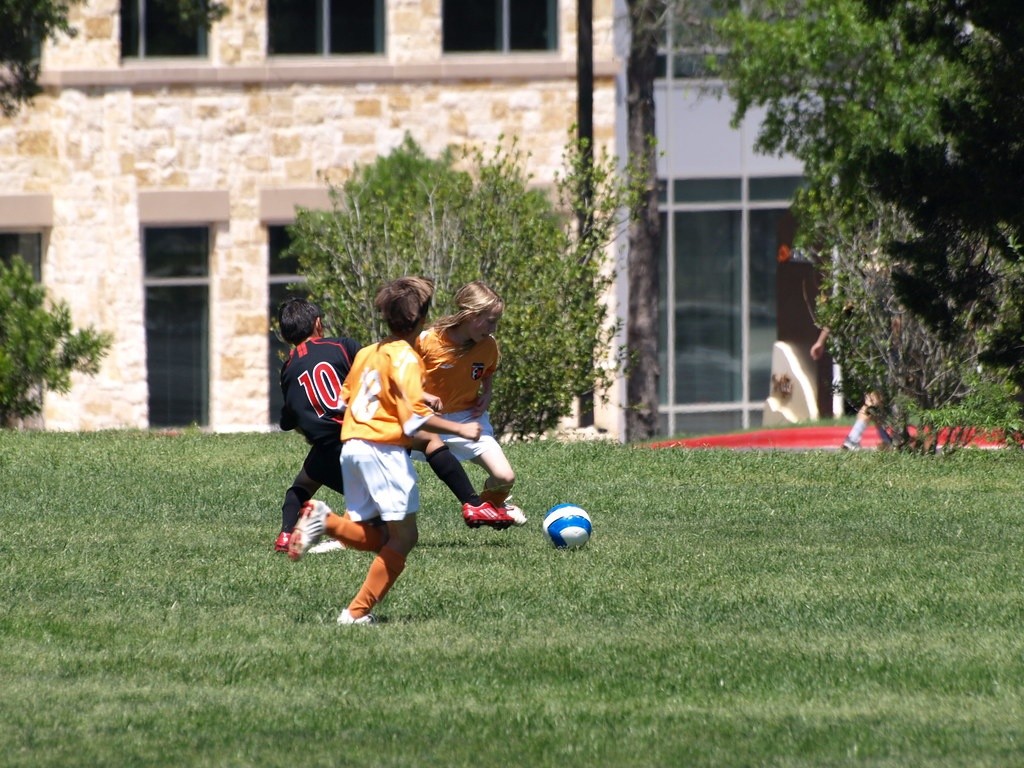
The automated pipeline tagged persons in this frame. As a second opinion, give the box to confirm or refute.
[811,326,902,450]
[288,276,483,625]
[276,298,527,552]
[410,280,528,526]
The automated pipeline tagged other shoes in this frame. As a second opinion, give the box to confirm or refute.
[842,435,861,450]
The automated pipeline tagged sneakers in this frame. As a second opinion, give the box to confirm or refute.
[337,609,376,625]
[289,499,332,562]
[461,501,515,530]
[499,495,528,527]
[274,530,291,553]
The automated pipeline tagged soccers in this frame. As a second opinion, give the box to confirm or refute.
[542,503,592,549]
